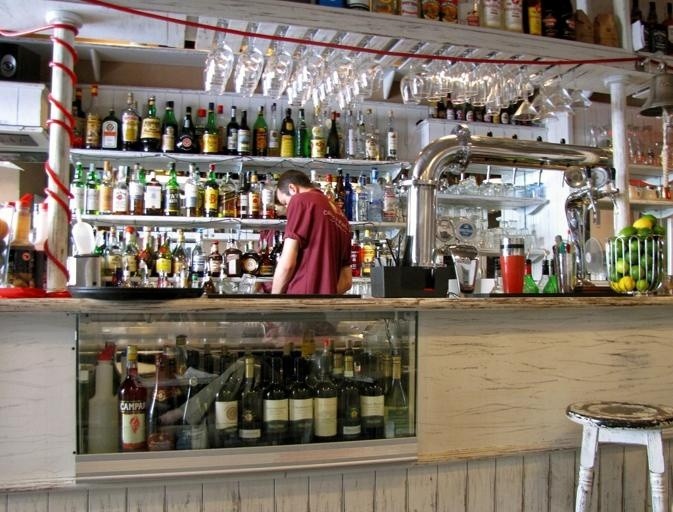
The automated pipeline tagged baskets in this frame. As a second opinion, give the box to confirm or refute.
[604,234,664,295]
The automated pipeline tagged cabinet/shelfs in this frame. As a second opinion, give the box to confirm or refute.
[66,146,417,293]
[625,160,672,210]
[418,115,552,293]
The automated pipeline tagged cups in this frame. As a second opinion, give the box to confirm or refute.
[553,252,577,293]
[499,237,525,293]
[434,204,530,250]
[488,260,504,294]
[439,176,544,201]
[453,258,477,297]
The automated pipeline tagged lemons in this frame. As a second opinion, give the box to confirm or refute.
[608,215,664,293]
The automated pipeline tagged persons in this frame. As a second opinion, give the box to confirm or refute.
[270,168,354,296]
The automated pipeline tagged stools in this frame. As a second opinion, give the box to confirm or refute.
[563,397,671,512]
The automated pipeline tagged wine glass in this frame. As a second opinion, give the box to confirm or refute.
[193,17,384,112]
[393,46,594,122]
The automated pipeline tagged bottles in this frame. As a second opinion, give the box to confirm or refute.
[0,196,48,290]
[389,0,628,48]
[87,337,406,450]
[67,159,410,222]
[70,84,404,161]
[589,121,672,206]
[78,223,399,274]
[525,258,557,293]
